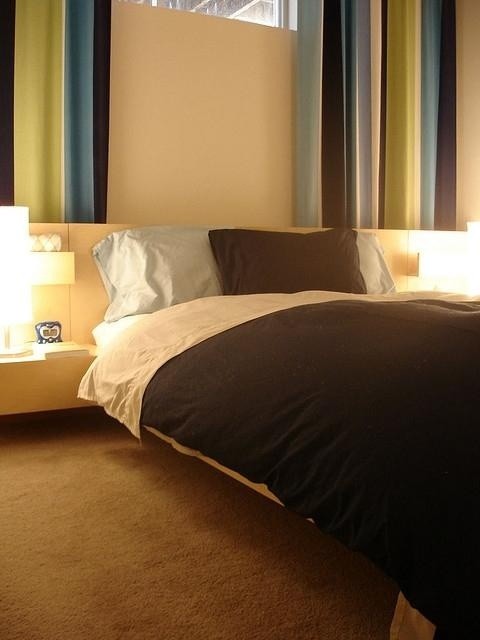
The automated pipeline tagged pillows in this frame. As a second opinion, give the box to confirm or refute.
[207,227,368,294]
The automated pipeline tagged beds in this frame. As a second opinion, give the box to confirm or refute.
[89,224,480,639]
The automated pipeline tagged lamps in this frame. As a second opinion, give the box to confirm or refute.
[0,206,33,358]
[31,251,75,286]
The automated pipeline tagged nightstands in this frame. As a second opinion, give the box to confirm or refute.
[0,342,98,415]
